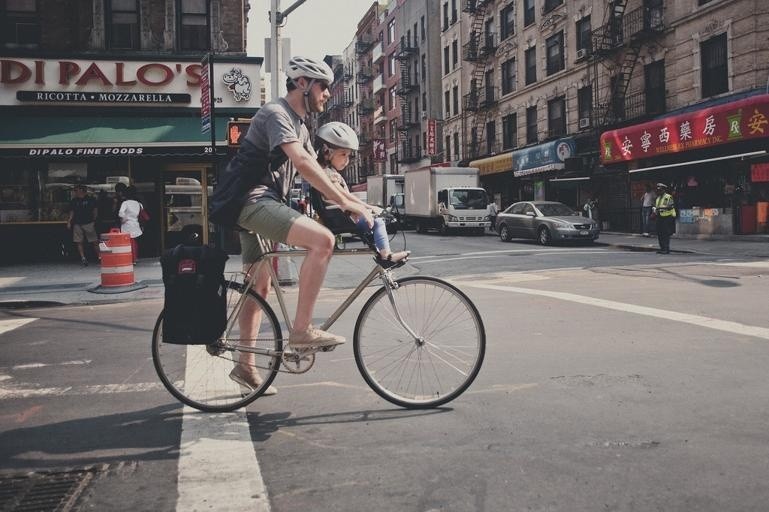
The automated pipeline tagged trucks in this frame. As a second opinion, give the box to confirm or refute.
[404,166,492,234]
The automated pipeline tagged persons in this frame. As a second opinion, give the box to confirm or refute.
[309,122,411,273]
[67,184,149,267]
[640,184,657,237]
[489,202,497,230]
[208,56,374,396]
[300,196,309,214]
[655,183,676,254]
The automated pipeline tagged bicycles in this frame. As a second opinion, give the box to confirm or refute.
[151,192,485,413]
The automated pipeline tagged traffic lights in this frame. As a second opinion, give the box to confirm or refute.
[227,121,251,148]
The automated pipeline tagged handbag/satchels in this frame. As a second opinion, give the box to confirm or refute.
[140,208,152,221]
[206,156,262,230]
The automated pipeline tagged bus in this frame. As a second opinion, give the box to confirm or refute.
[43,176,216,247]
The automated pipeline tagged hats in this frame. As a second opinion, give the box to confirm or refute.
[656,182,668,190]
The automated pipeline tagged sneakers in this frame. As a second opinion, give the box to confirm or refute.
[228,363,278,397]
[80,255,88,268]
[288,324,346,350]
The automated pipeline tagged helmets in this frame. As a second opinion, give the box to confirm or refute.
[284,55,335,86]
[313,121,362,151]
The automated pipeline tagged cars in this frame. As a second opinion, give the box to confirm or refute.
[288,173,405,235]
[495,200,601,246]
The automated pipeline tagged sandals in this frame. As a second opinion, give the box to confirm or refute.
[375,250,414,270]
